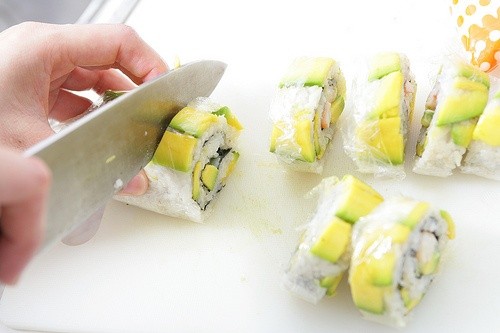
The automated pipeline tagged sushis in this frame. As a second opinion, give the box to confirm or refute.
[96,54,500,320]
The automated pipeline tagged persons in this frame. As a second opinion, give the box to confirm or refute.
[0,22,169,284]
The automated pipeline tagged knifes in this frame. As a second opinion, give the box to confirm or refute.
[21,59,228,257]
